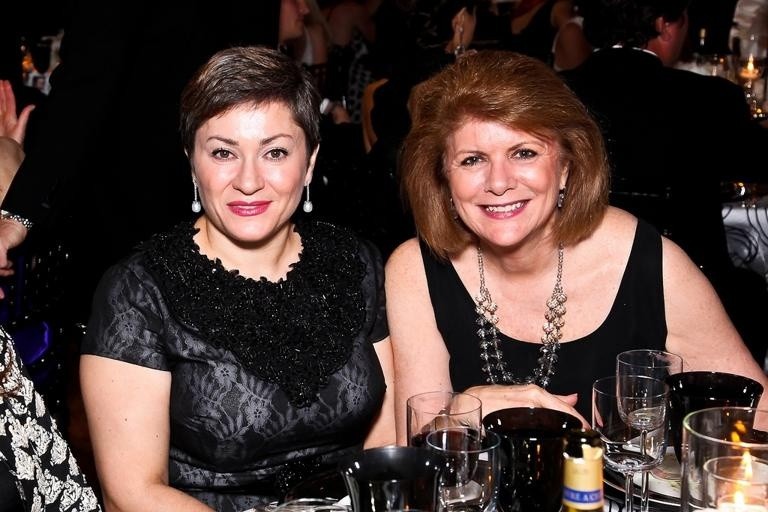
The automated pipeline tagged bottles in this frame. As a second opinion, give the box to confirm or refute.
[560,431,607,512]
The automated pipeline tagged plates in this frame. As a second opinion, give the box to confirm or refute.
[596,420,768,511]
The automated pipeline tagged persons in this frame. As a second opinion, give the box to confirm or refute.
[3,2,767,512]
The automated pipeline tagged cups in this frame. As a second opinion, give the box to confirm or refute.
[405,392,480,449]
[337,446,444,510]
[681,405,765,507]
[483,406,577,510]
[427,425,501,510]
[666,371,764,466]
[703,456,767,505]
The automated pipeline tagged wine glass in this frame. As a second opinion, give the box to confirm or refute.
[594,376,664,508]
[615,347,685,509]
